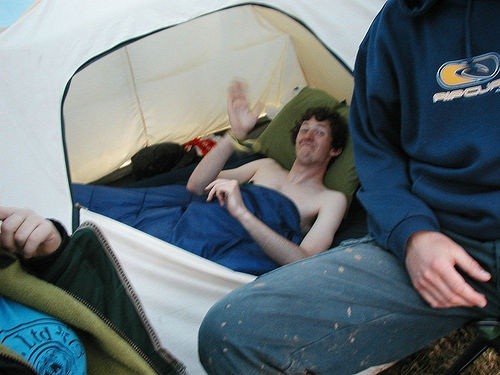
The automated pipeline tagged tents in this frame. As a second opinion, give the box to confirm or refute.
[0,2,409,375]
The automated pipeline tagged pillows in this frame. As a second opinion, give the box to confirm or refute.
[253,85,361,210]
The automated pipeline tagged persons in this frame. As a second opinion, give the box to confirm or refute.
[187,78,351,266]
[0,205,187,375]
[198,2,500,375]
